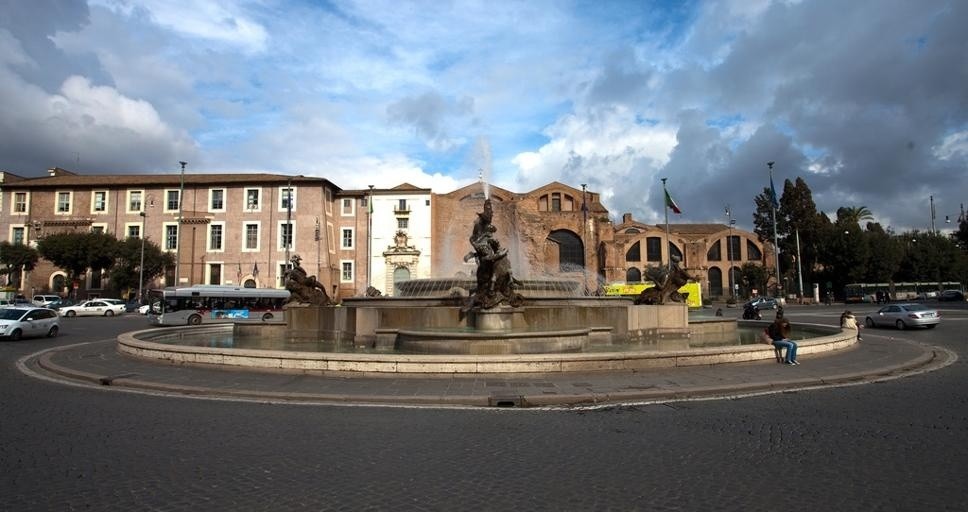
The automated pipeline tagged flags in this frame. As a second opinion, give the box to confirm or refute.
[770,169,780,209]
[664,187,682,214]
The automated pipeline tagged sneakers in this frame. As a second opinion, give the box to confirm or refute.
[792,359,800,365]
[785,360,795,366]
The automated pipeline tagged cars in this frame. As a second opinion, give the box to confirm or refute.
[938,290,964,303]
[0,306,61,341]
[14,293,162,317]
[866,302,941,330]
[744,296,778,310]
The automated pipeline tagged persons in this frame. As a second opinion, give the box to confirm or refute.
[744,289,760,318]
[168,298,289,310]
[919,290,925,304]
[840,309,864,341]
[876,288,890,304]
[776,306,784,320]
[768,310,800,366]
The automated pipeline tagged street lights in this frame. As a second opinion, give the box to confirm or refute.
[138,197,155,304]
[725,205,736,299]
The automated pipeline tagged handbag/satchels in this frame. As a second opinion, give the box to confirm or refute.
[761,328,774,345]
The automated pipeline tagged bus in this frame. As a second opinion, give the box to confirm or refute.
[602,282,702,311]
[845,282,963,303]
[146,284,292,325]
